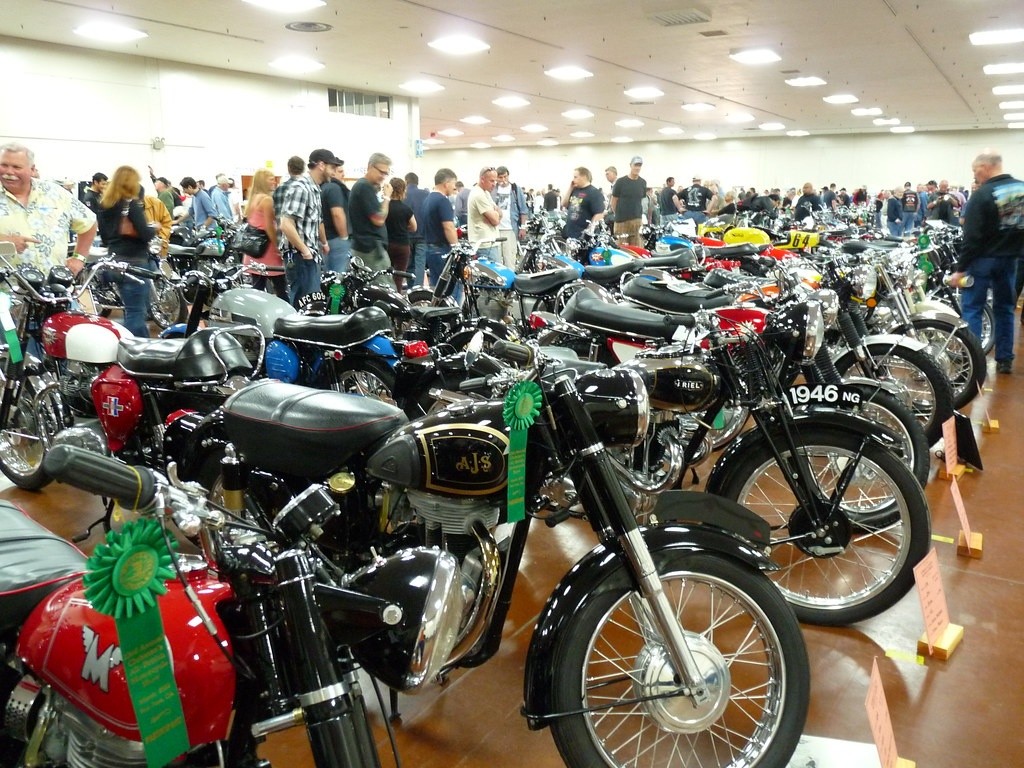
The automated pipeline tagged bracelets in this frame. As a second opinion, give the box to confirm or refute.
[341,236,348,240]
[301,251,310,256]
[321,242,328,247]
[381,194,390,202]
[517,224,527,231]
[72,253,87,263]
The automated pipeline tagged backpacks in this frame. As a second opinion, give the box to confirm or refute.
[903,193,917,212]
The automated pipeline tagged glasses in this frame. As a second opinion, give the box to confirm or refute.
[482,167,495,175]
[372,165,389,176]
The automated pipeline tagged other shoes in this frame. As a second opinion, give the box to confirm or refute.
[998,359,1011,373]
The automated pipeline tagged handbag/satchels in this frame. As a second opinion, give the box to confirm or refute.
[119,199,141,239]
[242,225,269,257]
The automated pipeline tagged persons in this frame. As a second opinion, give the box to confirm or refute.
[0,147,1024,376]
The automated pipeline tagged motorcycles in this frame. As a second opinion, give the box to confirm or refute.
[0,200,997,768]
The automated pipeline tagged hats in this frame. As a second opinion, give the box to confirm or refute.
[822,187,828,190]
[693,177,701,181]
[156,177,168,186]
[929,180,937,186]
[905,182,911,187]
[310,150,341,167]
[218,176,234,184]
[631,157,643,165]
